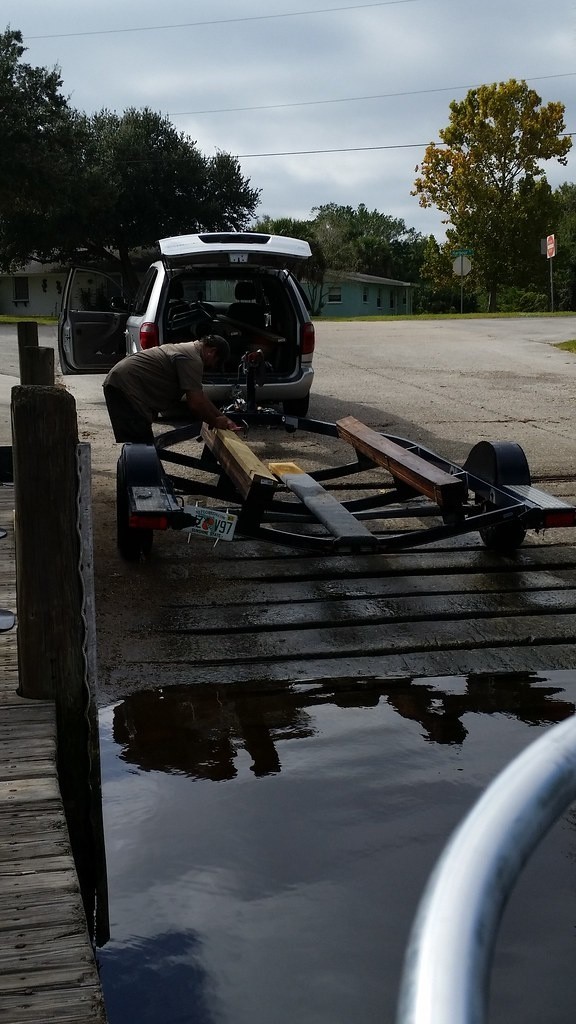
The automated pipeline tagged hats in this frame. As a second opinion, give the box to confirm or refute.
[211,335,230,375]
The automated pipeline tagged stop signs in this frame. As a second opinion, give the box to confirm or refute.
[547,234,555,258]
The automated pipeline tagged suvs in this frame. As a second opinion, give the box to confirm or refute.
[58,233,315,416]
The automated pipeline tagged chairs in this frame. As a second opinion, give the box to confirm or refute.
[166,281,189,324]
[229,283,266,328]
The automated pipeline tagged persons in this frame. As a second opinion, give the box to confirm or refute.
[102,334,238,446]
